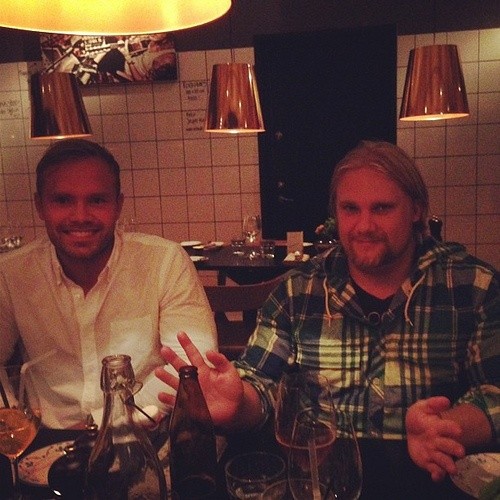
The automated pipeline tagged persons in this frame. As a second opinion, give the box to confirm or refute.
[0,137,220,436]
[152,140,499,481]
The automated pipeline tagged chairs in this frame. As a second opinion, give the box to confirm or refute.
[204,268,297,354]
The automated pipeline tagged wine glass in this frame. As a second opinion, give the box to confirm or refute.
[0,407,39,500]
[242,214,261,259]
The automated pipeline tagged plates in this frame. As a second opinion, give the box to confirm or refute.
[190,256,209,262]
[180,241,202,246]
[193,241,224,249]
[450,452,500,499]
[18,440,95,487]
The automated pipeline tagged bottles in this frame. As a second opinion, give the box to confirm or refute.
[48,427,98,500]
[84,354,168,500]
[167,365,219,500]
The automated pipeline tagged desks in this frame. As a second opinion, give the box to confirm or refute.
[185,245,318,285]
[0,429,500,499]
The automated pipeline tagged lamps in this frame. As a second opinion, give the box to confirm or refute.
[31,34,93,139]
[205,12,265,133]
[400,0,470,121]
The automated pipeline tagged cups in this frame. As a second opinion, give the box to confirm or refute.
[259,240,275,258]
[225,369,365,500]
[231,238,245,255]
